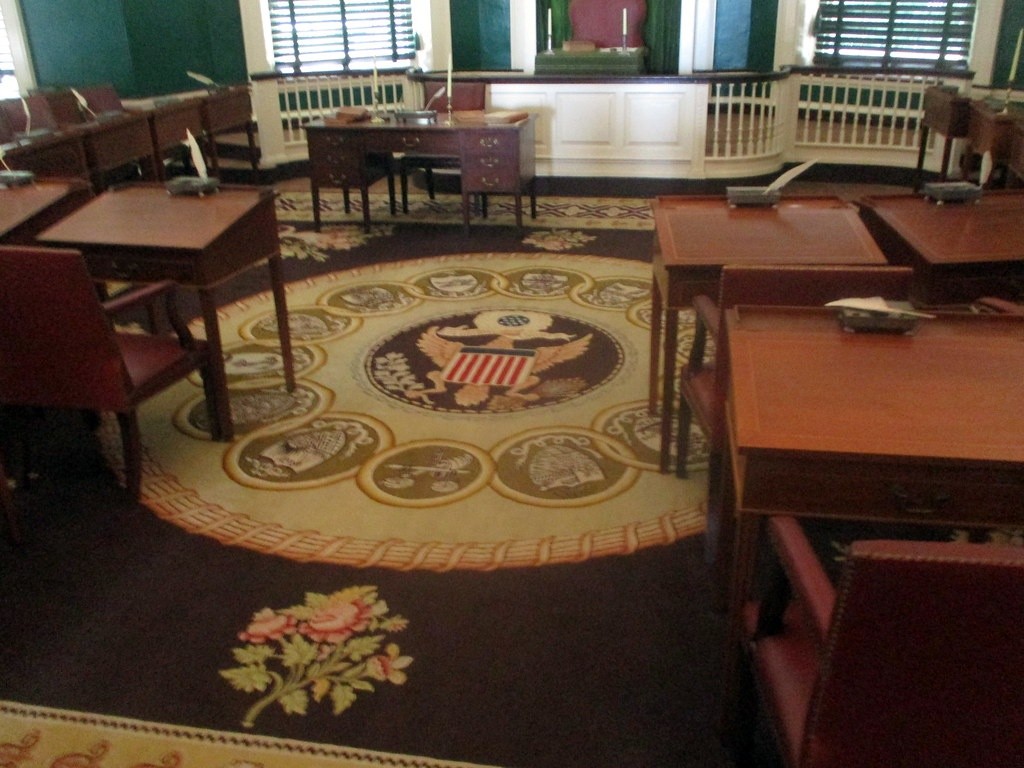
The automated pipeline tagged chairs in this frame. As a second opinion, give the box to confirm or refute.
[76,83,128,123]
[736,513,1024,768]
[568,0,647,49]
[399,80,490,223]
[0,106,22,149]
[0,246,223,545]
[673,262,916,483]
[43,90,86,132]
[0,93,62,138]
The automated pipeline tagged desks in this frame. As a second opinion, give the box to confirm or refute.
[0,81,297,442]
[534,47,647,76]
[646,85,1024,651]
[300,109,539,241]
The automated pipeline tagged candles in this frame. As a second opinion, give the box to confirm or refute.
[373,67,379,92]
[447,53,452,99]
[623,9,627,36]
[547,8,552,36]
[1008,28,1024,84]
